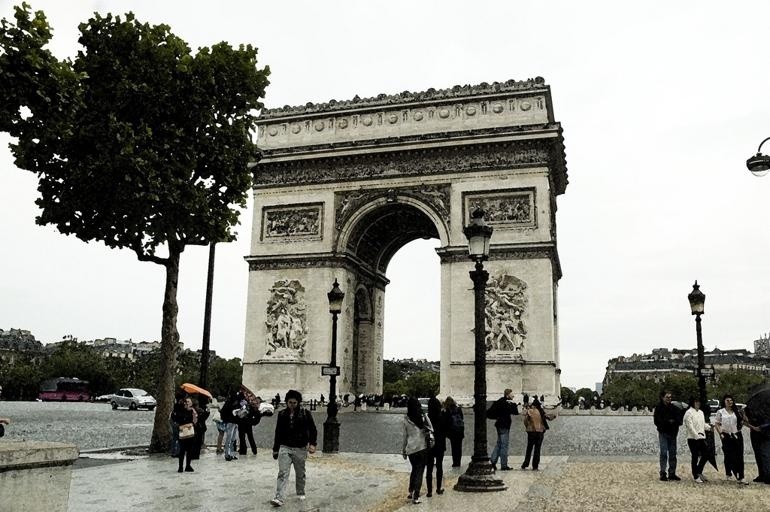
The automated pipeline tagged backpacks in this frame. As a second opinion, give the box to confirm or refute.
[486,398,504,419]
[448,407,464,434]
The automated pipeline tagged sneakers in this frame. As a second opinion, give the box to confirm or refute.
[296,494,306,501]
[737,479,749,485]
[694,477,703,482]
[178,467,183,472]
[270,498,284,506]
[184,466,194,471]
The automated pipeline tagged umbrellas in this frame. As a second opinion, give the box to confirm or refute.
[741,385,770,408]
[696,432,719,471]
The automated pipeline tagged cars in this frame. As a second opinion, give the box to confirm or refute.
[110,387,157,412]
[255,396,276,418]
[418,398,432,410]
[708,398,747,413]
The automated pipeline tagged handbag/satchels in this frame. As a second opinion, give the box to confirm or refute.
[177,423,195,439]
[423,413,435,449]
[195,415,207,435]
[248,404,261,426]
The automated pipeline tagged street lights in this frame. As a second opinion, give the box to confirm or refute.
[452,201,509,493]
[319,275,347,453]
[686,279,719,472]
[744,136,770,180]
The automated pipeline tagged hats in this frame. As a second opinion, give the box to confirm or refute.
[175,390,186,401]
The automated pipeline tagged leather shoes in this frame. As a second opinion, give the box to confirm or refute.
[407,489,444,504]
[492,463,497,470]
[501,463,513,470]
[753,476,764,482]
[668,475,681,480]
[659,476,670,481]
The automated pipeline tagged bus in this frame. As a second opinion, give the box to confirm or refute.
[38,375,92,402]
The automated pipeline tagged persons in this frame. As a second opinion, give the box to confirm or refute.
[0,416,11,437]
[270,389,317,506]
[714,394,750,486]
[443,396,464,467]
[271,392,281,409]
[262,281,307,357]
[483,273,527,350]
[401,397,435,504]
[653,388,684,482]
[682,395,713,484]
[740,395,770,486]
[168,382,261,472]
[485,388,519,470]
[521,397,550,471]
[342,393,408,408]
[426,397,449,497]
[523,393,545,408]
[420,187,449,215]
[320,393,324,408]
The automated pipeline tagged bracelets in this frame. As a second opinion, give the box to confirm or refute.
[719,431,723,435]
